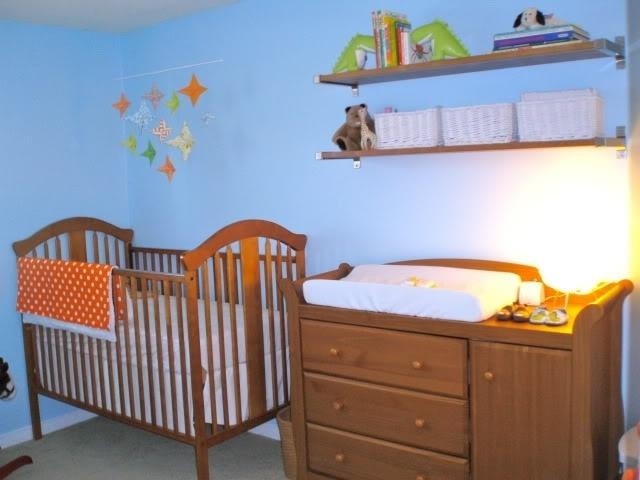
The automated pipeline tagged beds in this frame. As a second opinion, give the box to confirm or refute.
[11,216,307,479]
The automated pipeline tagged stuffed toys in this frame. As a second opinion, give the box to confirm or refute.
[357,109,377,151]
[512,8,567,30]
[331,104,375,150]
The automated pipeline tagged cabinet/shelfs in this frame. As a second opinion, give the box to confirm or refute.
[278,258,634,479]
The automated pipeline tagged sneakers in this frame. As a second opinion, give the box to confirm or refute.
[513,304,531,322]
[530,307,549,324]
[545,308,567,325]
[498,305,513,320]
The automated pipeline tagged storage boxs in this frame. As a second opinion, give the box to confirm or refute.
[440,102,517,147]
[518,88,602,141]
[373,108,441,149]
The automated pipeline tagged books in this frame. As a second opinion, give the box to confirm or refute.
[493,38,580,51]
[492,26,591,43]
[495,30,591,46]
[369,9,416,68]
